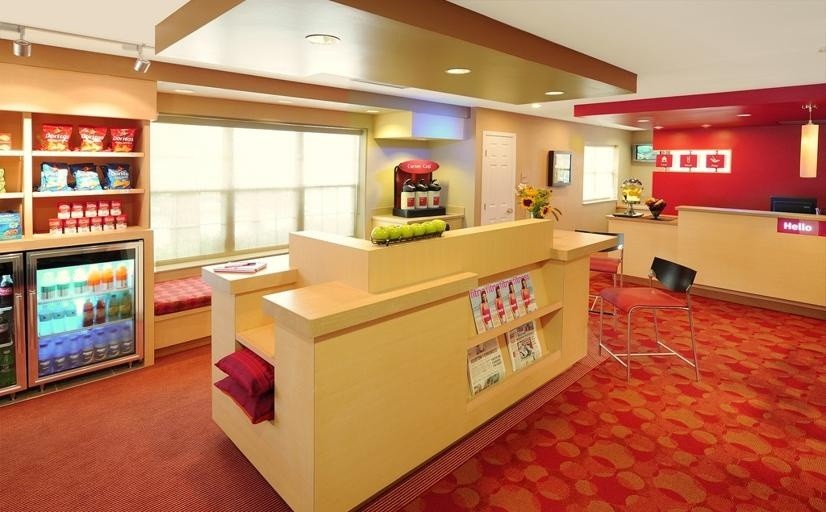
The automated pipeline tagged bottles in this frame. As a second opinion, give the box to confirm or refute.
[0,274,15,389]
[38,265,136,378]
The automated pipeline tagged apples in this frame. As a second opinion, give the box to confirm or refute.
[371,220,447,241]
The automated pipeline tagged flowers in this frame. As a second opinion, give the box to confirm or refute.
[519,184,563,222]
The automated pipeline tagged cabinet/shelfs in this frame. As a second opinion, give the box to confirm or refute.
[0,102,155,408]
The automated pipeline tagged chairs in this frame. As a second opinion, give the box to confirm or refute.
[574,229,701,385]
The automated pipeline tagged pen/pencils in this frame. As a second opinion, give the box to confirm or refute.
[225,262,256,268]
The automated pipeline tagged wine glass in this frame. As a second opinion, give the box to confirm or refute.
[647,204,666,220]
[621,199,640,216]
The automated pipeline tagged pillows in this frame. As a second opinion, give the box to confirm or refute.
[212,348,277,425]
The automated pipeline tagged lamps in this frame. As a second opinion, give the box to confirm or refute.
[798,104,821,177]
[11,29,33,57]
[134,44,151,75]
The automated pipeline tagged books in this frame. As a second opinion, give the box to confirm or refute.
[467,337,506,396]
[213,261,267,273]
[505,321,544,372]
[469,271,539,335]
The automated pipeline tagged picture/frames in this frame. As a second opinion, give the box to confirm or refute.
[547,150,572,186]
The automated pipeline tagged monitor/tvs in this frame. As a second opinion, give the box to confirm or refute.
[632,143,656,162]
[770,197,817,215]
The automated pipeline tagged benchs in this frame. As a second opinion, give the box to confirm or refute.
[153,276,212,356]
